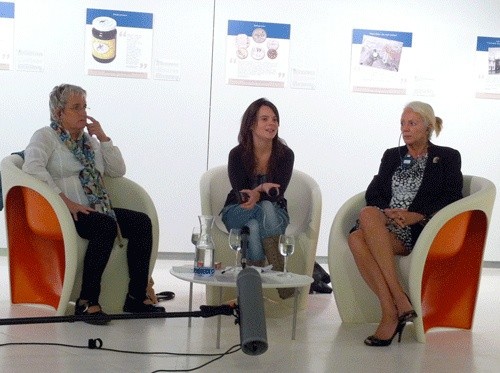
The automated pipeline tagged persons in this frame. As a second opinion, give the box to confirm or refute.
[21,84,166,326]
[217,97,298,299]
[347,101,464,347]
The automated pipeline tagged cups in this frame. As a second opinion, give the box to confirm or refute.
[190,227,200,242]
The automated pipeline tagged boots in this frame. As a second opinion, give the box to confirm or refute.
[246,259,265,272]
[262,237,295,300]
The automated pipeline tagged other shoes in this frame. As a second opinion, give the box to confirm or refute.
[123,293,165,313]
[75,298,111,323]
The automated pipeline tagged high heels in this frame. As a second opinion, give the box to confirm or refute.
[364,317,406,347]
[394,292,418,323]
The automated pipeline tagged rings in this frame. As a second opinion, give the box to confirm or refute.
[90,125,95,127]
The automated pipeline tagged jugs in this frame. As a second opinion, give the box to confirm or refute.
[197,215,214,275]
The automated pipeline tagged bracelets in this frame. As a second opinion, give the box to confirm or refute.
[259,183,263,193]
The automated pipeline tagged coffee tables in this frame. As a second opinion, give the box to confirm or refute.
[170,267,314,349]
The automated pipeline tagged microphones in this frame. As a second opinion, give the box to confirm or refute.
[269,187,278,195]
[239,226,250,269]
[237,268,268,355]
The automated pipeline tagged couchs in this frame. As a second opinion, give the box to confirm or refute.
[199,165,322,310]
[328,175,497,344]
[0,155,160,317]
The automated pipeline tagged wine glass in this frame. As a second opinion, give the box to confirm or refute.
[279,234,295,279]
[228,229,243,275]
